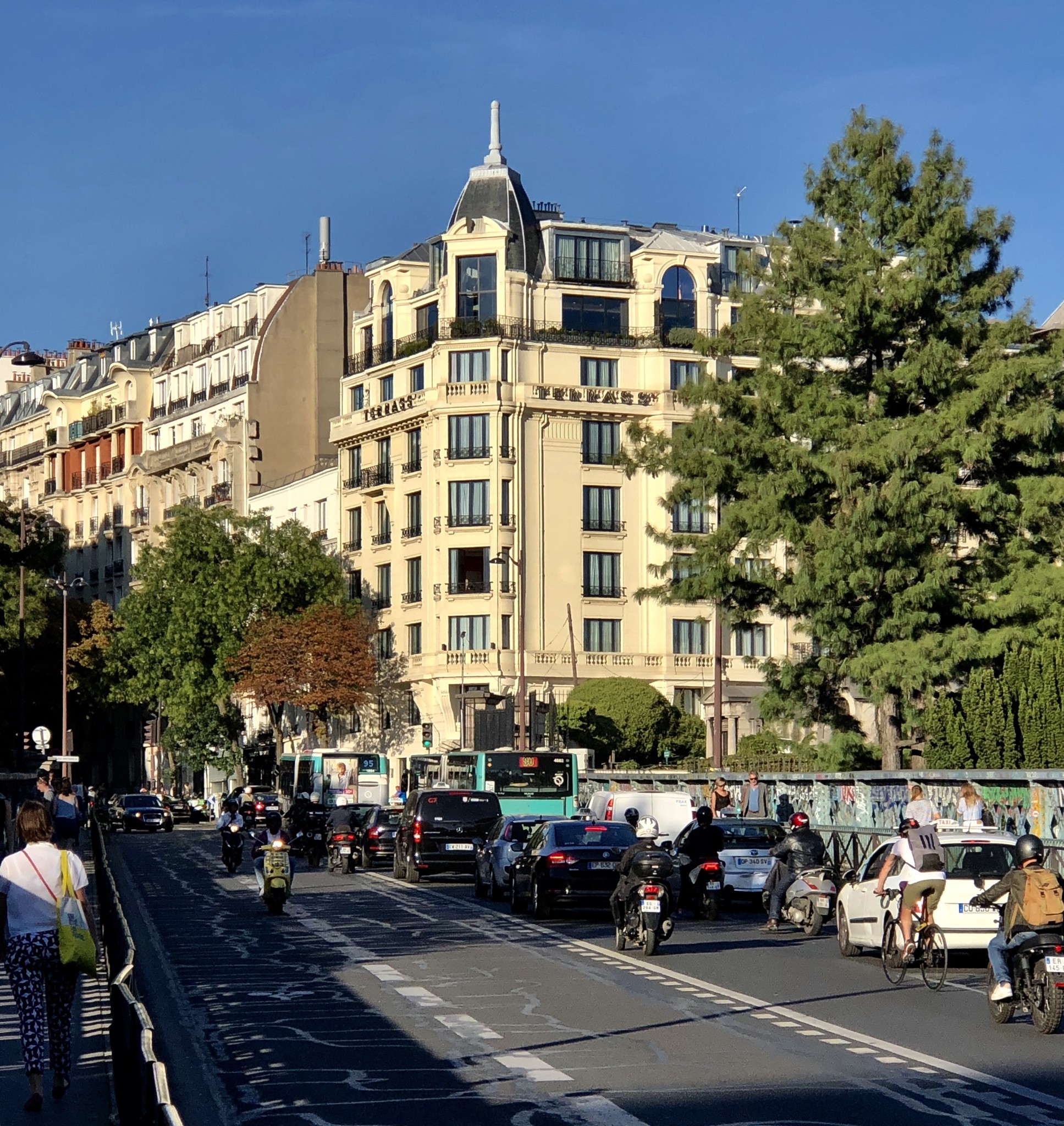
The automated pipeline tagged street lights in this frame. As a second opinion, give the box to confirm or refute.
[44,571,89,790]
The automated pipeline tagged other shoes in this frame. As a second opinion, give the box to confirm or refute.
[50,1076,71,1099]
[26,1094,43,1113]
[990,983,1012,1000]
[759,920,778,931]
[258,888,264,897]
[902,942,915,963]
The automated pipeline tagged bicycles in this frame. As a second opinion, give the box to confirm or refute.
[874,887,948,993]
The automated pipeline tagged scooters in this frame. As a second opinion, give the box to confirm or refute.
[324,824,359,874]
[687,856,727,921]
[249,830,304,917]
[218,821,249,874]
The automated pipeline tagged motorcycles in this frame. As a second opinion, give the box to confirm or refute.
[974,874,1064,1035]
[779,865,836,937]
[609,840,674,957]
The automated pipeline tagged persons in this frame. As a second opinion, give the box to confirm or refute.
[0,766,1064,1114]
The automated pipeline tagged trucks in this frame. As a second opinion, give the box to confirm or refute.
[584,790,697,845]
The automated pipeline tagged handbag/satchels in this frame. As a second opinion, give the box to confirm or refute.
[57,850,97,967]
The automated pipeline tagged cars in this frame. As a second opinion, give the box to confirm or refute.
[107,793,175,833]
[153,784,406,870]
[662,807,788,916]
[155,794,192,826]
[471,814,570,903]
[836,817,1019,969]
[510,807,643,920]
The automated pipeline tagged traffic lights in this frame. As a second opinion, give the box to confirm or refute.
[422,722,434,749]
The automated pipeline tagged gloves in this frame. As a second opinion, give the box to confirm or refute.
[970,896,979,907]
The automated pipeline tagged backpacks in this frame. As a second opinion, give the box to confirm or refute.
[1012,868,1064,926]
[903,824,944,872]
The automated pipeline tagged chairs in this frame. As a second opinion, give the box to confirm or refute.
[377,814,389,822]
[962,852,991,872]
[744,828,757,836]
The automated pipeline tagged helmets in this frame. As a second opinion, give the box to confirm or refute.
[294,794,305,802]
[899,819,918,838]
[635,818,658,839]
[1015,834,1043,866]
[788,811,809,830]
[336,797,347,806]
[696,806,713,824]
[302,792,309,799]
[309,792,320,802]
[265,812,282,830]
[243,787,252,794]
[227,799,239,811]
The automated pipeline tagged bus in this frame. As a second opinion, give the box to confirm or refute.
[272,747,390,806]
[400,749,580,817]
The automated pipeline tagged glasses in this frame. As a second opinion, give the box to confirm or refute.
[750,777,758,780]
[718,784,725,787]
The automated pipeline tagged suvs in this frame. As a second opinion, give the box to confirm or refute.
[393,781,503,884]
